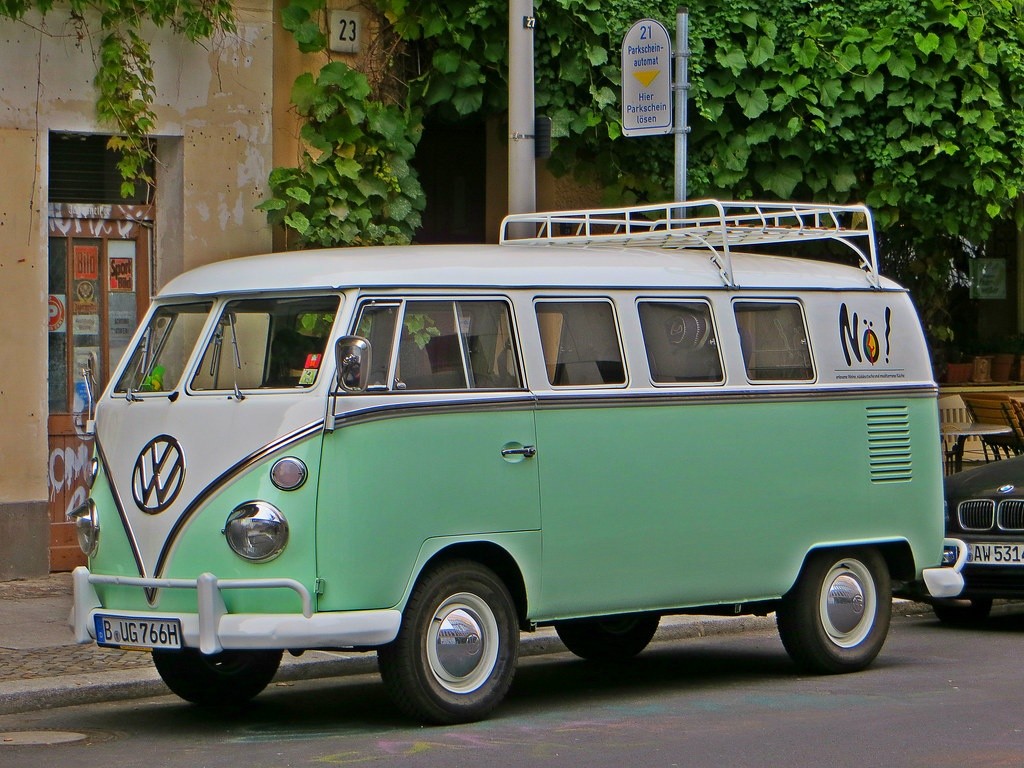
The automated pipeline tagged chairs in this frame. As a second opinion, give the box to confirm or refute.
[937,392,1024,476]
[426,332,491,387]
[267,330,323,388]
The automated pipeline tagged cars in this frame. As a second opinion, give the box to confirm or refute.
[932,453,1024,629]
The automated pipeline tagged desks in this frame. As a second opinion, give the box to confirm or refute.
[941,423,1013,476]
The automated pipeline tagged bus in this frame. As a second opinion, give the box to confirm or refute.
[65,197,942,727]
